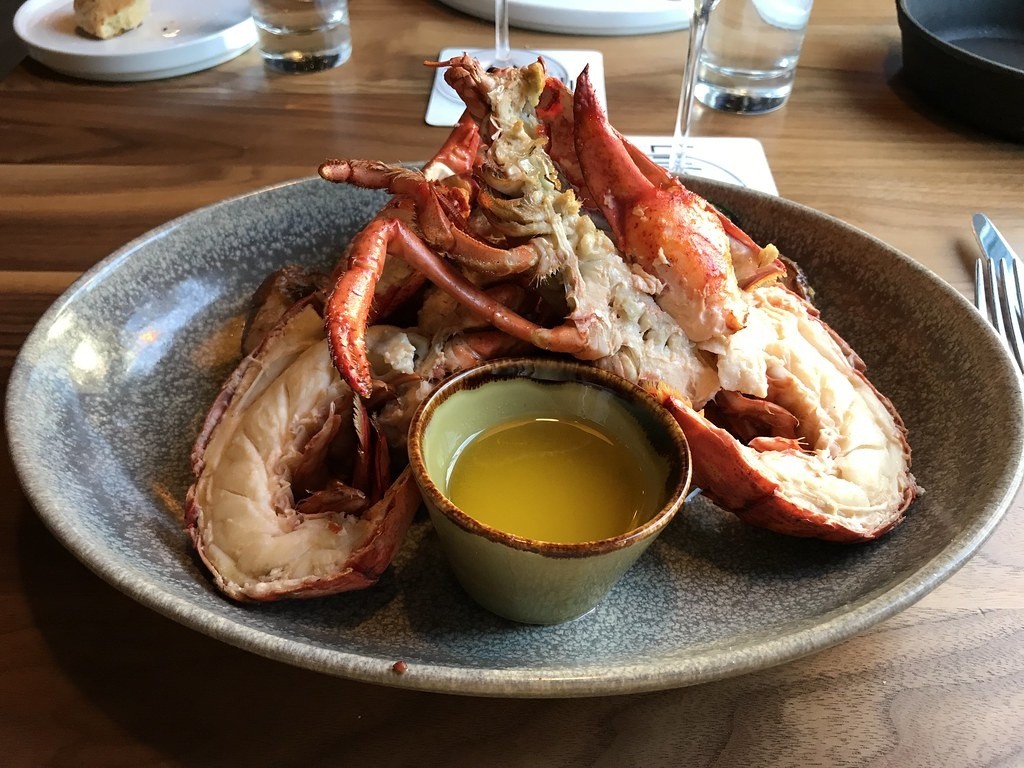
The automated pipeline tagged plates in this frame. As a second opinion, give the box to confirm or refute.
[441,0,689,36]
[5,160,1023,699]
[13,0,258,81]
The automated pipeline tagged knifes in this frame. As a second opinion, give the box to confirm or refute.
[970,213,1023,313]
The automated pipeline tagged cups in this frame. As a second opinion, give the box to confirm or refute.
[252,0,352,75]
[406,360,693,626]
[694,1,814,113]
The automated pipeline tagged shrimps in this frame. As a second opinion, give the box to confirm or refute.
[172,54,919,602]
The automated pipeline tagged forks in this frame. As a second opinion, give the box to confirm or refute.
[975,258,1023,382]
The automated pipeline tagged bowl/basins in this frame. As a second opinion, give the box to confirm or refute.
[895,1,1024,141]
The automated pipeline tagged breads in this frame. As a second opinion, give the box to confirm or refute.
[72,0,152,40]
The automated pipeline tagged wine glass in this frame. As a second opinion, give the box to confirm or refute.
[626,1,745,190]
[432,0,567,106]
